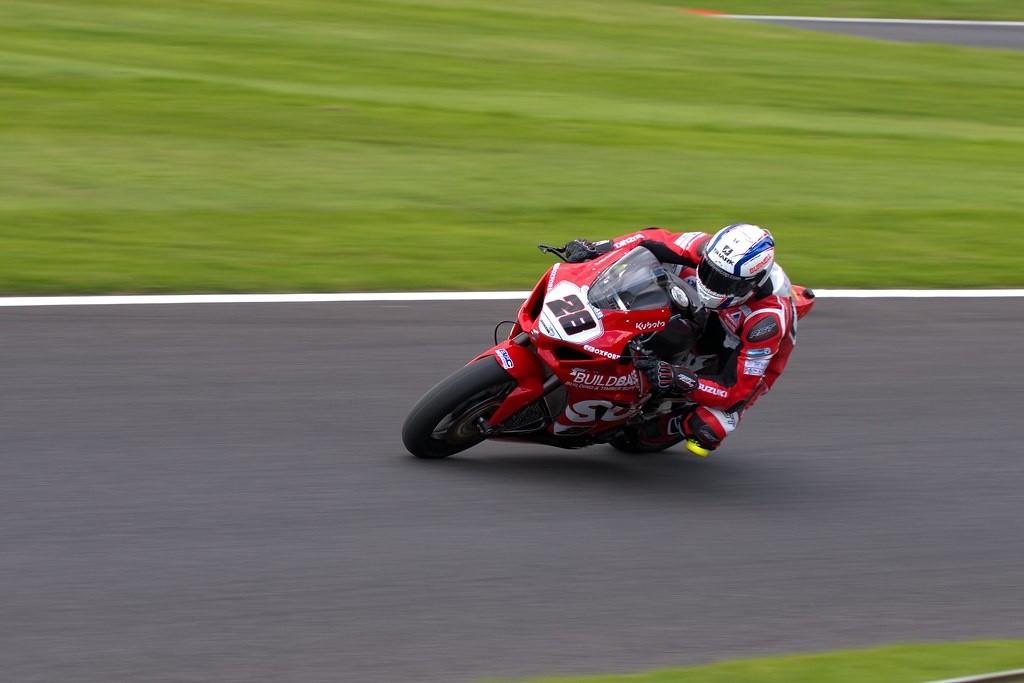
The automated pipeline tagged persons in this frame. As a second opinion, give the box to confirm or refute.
[565,222,799,457]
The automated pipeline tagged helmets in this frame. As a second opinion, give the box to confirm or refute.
[698,223,775,310]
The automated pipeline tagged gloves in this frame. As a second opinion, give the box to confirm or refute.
[566,239,614,262]
[635,360,698,393]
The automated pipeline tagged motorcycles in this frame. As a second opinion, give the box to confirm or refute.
[403,245,816,458]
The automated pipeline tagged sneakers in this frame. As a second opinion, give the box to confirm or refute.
[638,408,692,447]
